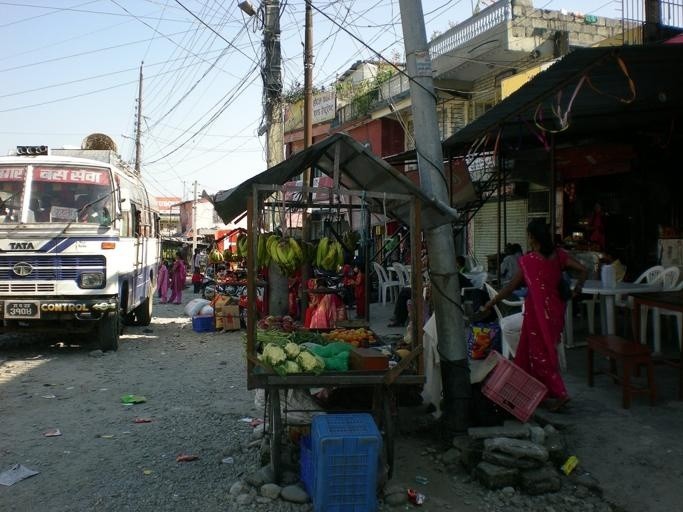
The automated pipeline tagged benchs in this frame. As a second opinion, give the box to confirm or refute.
[587,334,658,410]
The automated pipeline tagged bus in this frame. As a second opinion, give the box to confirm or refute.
[0,145,161,351]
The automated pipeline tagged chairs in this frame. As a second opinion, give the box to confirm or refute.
[485,282,569,374]
[372,255,499,305]
[564,251,607,347]
[614,264,682,356]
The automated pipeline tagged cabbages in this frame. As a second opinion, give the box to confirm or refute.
[263,342,325,376]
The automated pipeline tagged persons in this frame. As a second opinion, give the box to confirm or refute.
[386,287,412,328]
[157,246,211,305]
[501,243,524,293]
[136,210,141,236]
[486,217,589,414]
[348,263,368,320]
[455,255,470,276]
[304,278,347,330]
[1,193,111,224]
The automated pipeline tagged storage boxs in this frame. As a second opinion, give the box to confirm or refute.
[350,346,388,370]
[478,357,547,423]
[221,306,241,331]
[213,295,231,312]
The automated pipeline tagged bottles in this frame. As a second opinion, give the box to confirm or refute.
[600,264,617,290]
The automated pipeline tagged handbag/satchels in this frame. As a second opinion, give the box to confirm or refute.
[557,279,572,300]
[468,323,502,359]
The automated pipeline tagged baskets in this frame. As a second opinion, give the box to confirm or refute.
[481,351,549,422]
[192,316,216,332]
[309,414,383,512]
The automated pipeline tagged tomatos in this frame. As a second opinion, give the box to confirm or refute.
[321,327,374,347]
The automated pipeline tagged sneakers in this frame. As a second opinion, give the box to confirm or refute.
[550,397,570,412]
[387,321,404,327]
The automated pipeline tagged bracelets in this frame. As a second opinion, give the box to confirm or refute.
[577,282,585,288]
[486,301,492,308]
[573,288,581,295]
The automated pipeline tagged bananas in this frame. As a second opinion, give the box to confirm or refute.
[238,233,345,271]
